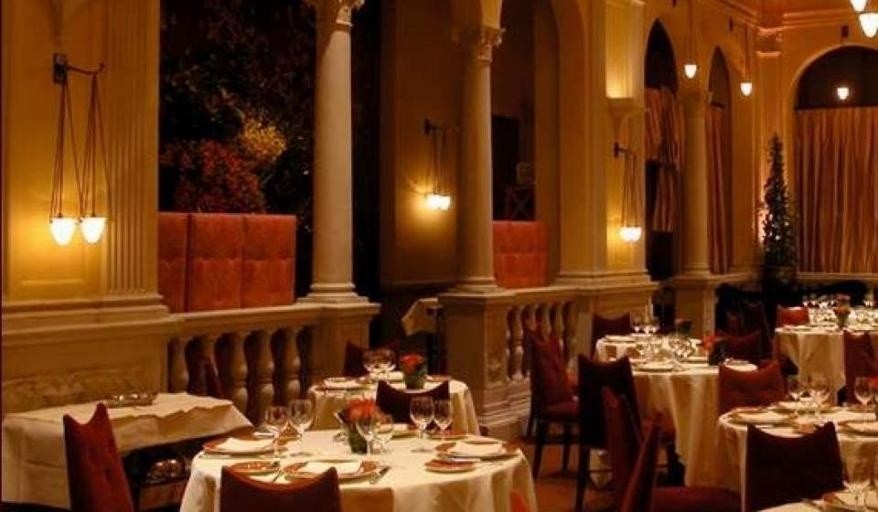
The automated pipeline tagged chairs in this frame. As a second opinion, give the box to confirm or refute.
[713,360,784,417]
[62,403,138,511]
[576,352,655,510]
[340,337,399,380]
[774,301,877,405]
[522,328,582,480]
[220,464,343,512]
[375,379,450,428]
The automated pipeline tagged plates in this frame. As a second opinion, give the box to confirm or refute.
[200,435,283,454]
[423,427,506,473]
[228,460,281,474]
[280,457,380,480]
[728,400,878,436]
[318,372,453,390]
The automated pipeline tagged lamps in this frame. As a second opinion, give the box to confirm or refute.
[45,52,107,247]
[612,144,641,243]
[424,121,450,212]
[670,1,698,80]
[835,25,850,101]
[727,18,754,97]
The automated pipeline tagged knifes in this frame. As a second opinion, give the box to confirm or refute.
[370,465,392,484]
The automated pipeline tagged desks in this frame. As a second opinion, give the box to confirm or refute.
[180,424,537,512]
[2,391,254,510]
[595,333,767,465]
[716,397,876,512]
[305,375,483,436]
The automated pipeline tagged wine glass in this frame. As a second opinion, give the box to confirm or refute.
[352,412,393,460]
[286,398,317,459]
[411,395,454,445]
[264,403,288,458]
[840,452,878,511]
[802,291,875,328]
[629,310,693,364]
[785,372,878,423]
[362,344,396,384]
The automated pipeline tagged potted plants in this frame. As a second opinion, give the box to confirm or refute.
[754,132,802,289]
